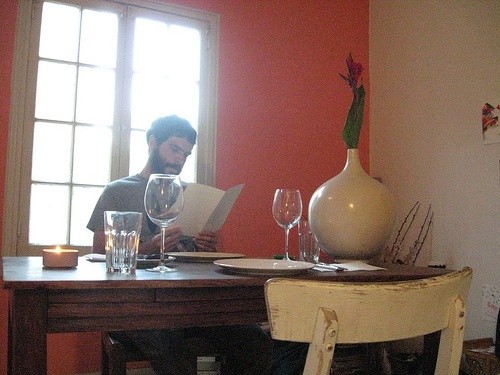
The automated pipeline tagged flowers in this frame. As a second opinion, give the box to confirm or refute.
[338,49,367,148]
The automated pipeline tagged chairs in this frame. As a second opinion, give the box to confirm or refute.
[264,265,474,375]
[101,331,147,375]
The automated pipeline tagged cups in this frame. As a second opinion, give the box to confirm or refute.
[103,211,143,276]
[296,220,321,262]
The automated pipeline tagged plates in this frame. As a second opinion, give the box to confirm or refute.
[165,251,247,263]
[212,257,314,276]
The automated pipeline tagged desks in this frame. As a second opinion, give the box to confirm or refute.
[0,256,457,375]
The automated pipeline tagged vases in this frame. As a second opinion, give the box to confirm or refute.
[308,149,397,262]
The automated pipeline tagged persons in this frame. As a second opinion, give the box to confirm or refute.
[87,115,273,375]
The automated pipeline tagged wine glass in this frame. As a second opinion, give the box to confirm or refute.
[144,172,185,273]
[271,189,303,261]
